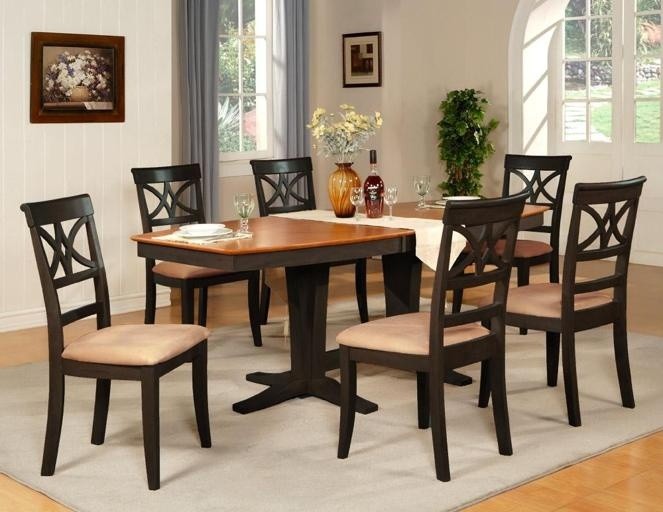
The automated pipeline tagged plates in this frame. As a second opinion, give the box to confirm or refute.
[177,222,233,237]
[435,196,480,209]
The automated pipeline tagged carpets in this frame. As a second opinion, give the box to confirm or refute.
[0,288,662,511]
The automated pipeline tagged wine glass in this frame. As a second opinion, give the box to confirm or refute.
[348,185,363,223]
[383,186,399,223]
[414,175,431,210]
[234,193,255,237]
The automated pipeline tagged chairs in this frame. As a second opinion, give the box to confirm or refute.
[130,163,262,361]
[17,193,212,493]
[450,152,572,333]
[334,191,531,481]
[249,154,368,338]
[475,175,648,427]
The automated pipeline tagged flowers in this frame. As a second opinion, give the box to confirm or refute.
[42,48,112,105]
[307,103,383,162]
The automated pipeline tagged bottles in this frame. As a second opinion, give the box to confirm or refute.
[363,150,384,219]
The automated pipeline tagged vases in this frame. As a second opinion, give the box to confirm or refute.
[327,160,362,217]
[66,85,91,102]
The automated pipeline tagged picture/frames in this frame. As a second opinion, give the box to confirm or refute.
[340,32,382,89]
[28,30,125,125]
